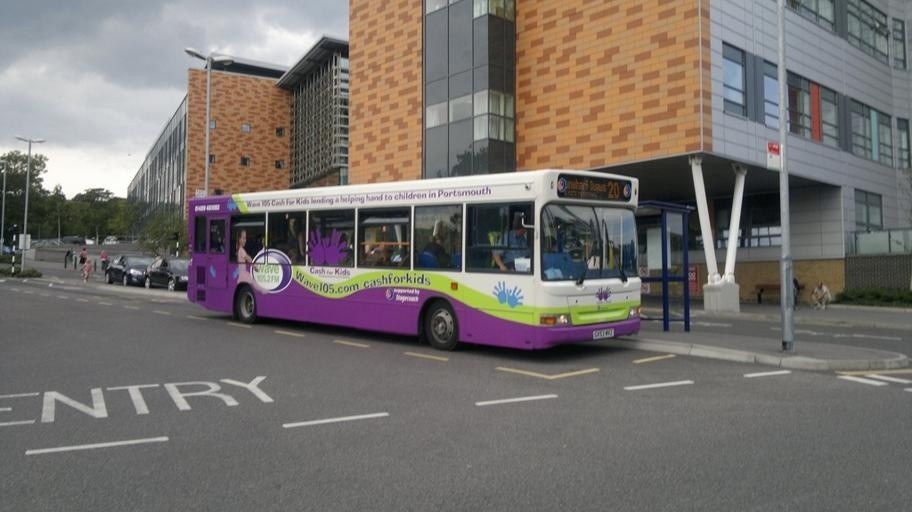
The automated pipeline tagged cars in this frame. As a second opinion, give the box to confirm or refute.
[142,255,189,292]
[64,234,119,247]
[105,253,156,287]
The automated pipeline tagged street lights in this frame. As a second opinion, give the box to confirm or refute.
[15,135,46,273]
[184,47,234,195]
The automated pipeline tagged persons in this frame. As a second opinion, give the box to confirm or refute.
[810,279,832,311]
[491,219,531,273]
[236,228,270,295]
[79,247,108,283]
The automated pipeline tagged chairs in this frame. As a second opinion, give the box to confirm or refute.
[418,251,440,268]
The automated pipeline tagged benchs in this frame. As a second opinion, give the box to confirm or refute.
[749,283,805,305]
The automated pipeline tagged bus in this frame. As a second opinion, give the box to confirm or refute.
[188,168,643,356]
